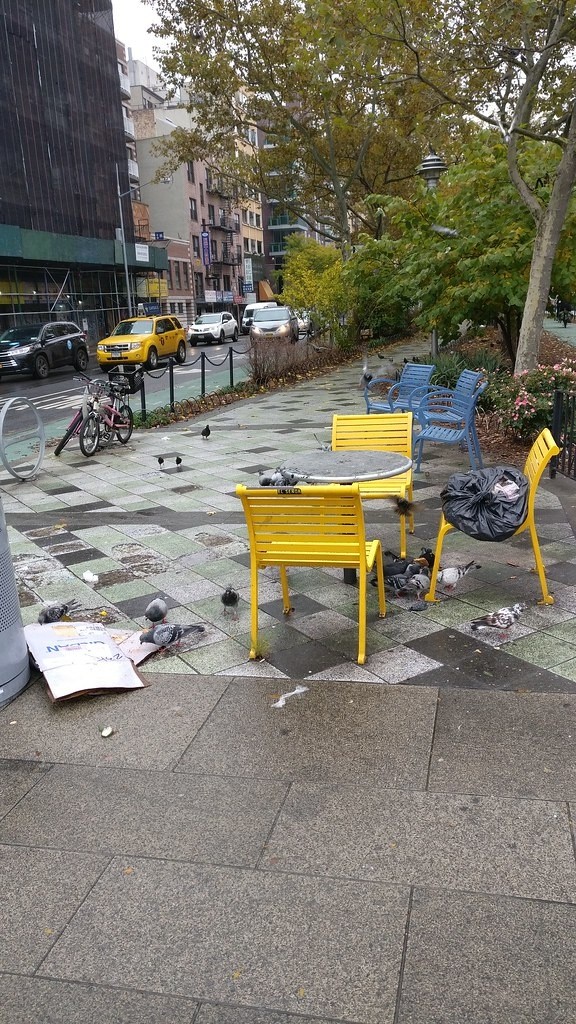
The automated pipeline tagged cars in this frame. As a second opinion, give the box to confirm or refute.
[250,308,309,348]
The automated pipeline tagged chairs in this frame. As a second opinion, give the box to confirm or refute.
[408,369,482,448]
[321,412,412,559]
[236,483,386,664]
[412,381,488,471]
[364,363,435,413]
[426,428,559,604]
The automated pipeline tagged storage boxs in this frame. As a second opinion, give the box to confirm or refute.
[108,364,143,394]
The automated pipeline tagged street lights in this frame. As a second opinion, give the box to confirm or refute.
[115,162,175,317]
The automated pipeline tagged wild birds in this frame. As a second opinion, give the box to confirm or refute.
[404,358,409,364]
[176,456,182,466]
[157,457,164,466]
[412,357,415,362]
[416,357,420,362]
[201,425,210,439]
[378,354,384,359]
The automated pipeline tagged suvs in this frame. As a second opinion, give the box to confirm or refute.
[0,322,90,377]
[95,313,189,368]
[186,312,239,343]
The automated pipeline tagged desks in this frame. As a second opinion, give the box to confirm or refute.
[280,450,412,583]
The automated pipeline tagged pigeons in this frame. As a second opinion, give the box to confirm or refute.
[436,560,476,592]
[221,586,240,621]
[144,596,168,629]
[369,547,442,601]
[259,466,310,486]
[38,598,83,626]
[139,623,205,654]
[470,603,529,631]
[388,495,423,517]
[313,433,332,450]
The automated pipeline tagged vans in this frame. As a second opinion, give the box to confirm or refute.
[242,301,278,334]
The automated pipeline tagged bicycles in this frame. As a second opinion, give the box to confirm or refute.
[53,364,144,459]
